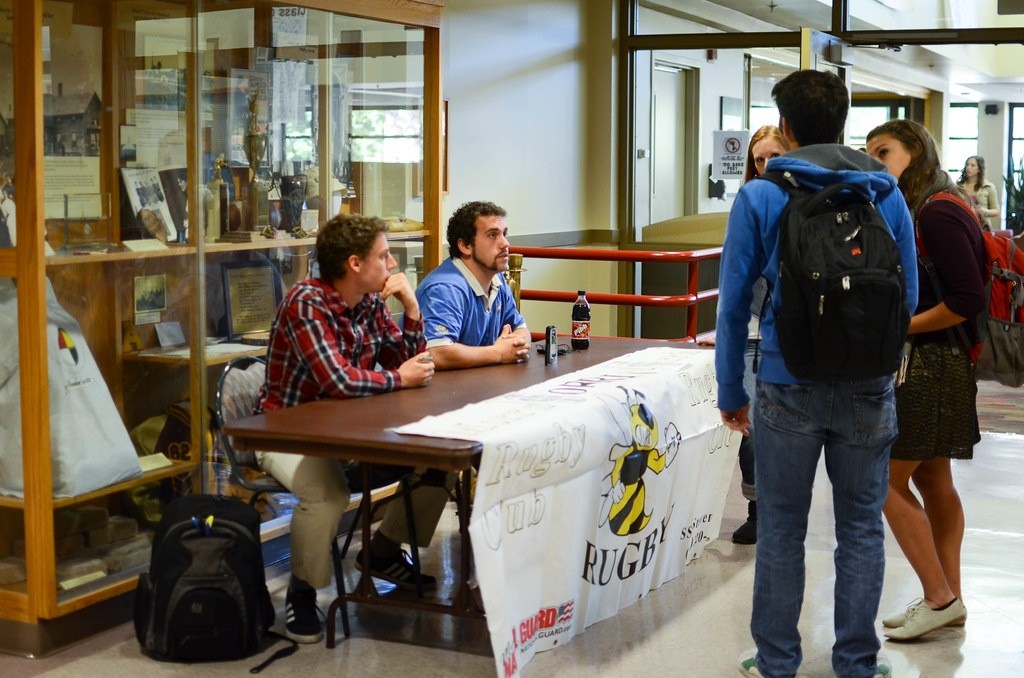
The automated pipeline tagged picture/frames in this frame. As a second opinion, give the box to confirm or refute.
[221,258,282,345]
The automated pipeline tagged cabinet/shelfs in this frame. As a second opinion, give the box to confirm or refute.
[0,0,449,662]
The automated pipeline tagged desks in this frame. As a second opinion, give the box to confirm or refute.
[221,338,700,651]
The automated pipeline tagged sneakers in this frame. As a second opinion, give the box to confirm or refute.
[738,649,767,678]
[354,544,437,592]
[285,584,328,644]
[873,653,892,678]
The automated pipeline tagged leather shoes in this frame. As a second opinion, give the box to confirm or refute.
[883,598,965,640]
[882,598,967,628]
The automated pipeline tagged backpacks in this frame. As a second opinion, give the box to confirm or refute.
[914,193,1024,388]
[133,494,276,663]
[755,169,911,381]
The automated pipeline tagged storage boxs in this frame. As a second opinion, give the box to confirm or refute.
[300,209,319,234]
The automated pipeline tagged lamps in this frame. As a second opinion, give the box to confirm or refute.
[985,104,998,115]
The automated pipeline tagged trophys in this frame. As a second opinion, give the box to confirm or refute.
[205,152,232,243]
[215,86,271,242]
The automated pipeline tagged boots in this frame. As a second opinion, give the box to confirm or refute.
[732,501,758,544]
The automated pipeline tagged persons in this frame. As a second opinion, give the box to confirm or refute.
[255,214,459,645]
[385,201,531,472]
[716,71,918,678]
[177,174,188,230]
[1,175,17,247]
[731,125,790,545]
[956,156,1000,233]
[866,119,992,641]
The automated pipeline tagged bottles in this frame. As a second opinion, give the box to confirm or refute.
[571,290,591,350]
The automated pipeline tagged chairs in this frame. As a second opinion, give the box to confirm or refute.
[214,355,422,639]
